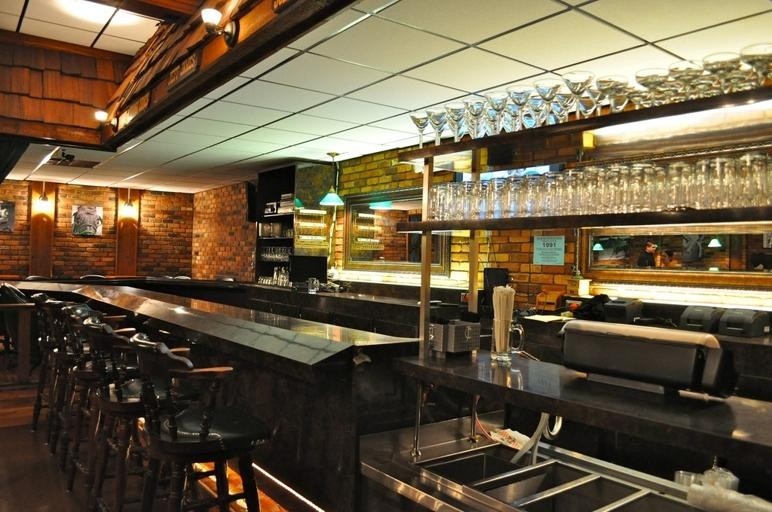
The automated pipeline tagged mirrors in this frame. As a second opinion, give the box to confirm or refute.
[578,124,772,291]
[342,183,451,275]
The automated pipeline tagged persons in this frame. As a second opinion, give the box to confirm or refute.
[751,240,770,271]
[638,241,657,269]
[659,249,674,267]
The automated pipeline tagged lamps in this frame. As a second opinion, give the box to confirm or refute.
[95,110,118,132]
[124,188,133,207]
[39,181,48,201]
[319,152,345,206]
[368,200,393,210]
[201,8,239,47]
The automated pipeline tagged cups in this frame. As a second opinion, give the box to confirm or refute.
[489,318,525,363]
[308,277,319,295]
[427,150,772,220]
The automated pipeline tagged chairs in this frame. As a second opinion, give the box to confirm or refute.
[28,288,190,509]
[121,331,264,512]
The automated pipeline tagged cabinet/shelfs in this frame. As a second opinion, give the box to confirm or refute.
[248,161,331,286]
[393,84,772,234]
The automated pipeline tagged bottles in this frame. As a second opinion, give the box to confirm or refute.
[257,265,293,290]
[259,245,293,263]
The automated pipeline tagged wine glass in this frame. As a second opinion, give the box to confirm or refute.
[411,70,631,148]
[628,42,771,112]
